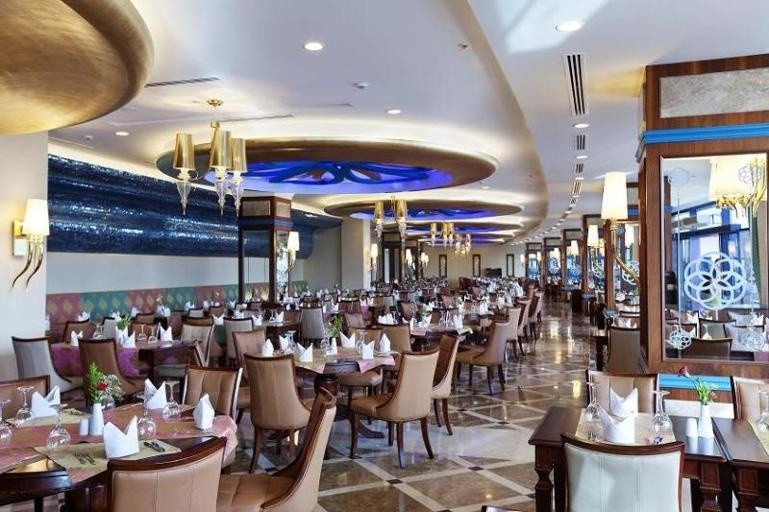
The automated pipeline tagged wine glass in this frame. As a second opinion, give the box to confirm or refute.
[261,331,396,365]
[0,379,182,464]
[572,379,675,444]
[185,276,523,332]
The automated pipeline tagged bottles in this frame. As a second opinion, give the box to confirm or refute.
[71,320,180,347]
[685,436,697,454]
[684,416,697,437]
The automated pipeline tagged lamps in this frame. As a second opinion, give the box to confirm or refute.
[12,198,51,289]
[374,194,408,244]
[420,251,430,267]
[430,222,455,248]
[284,230,300,274]
[454,233,473,255]
[519,170,641,295]
[367,243,379,272]
[706,151,768,220]
[172,99,249,218]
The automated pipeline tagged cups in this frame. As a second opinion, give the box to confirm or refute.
[758,388,768,427]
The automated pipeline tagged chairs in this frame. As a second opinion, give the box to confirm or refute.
[214,387,337,512]
[1,376,51,420]
[528,273,769,512]
[107,436,228,512]
[10,275,543,467]
